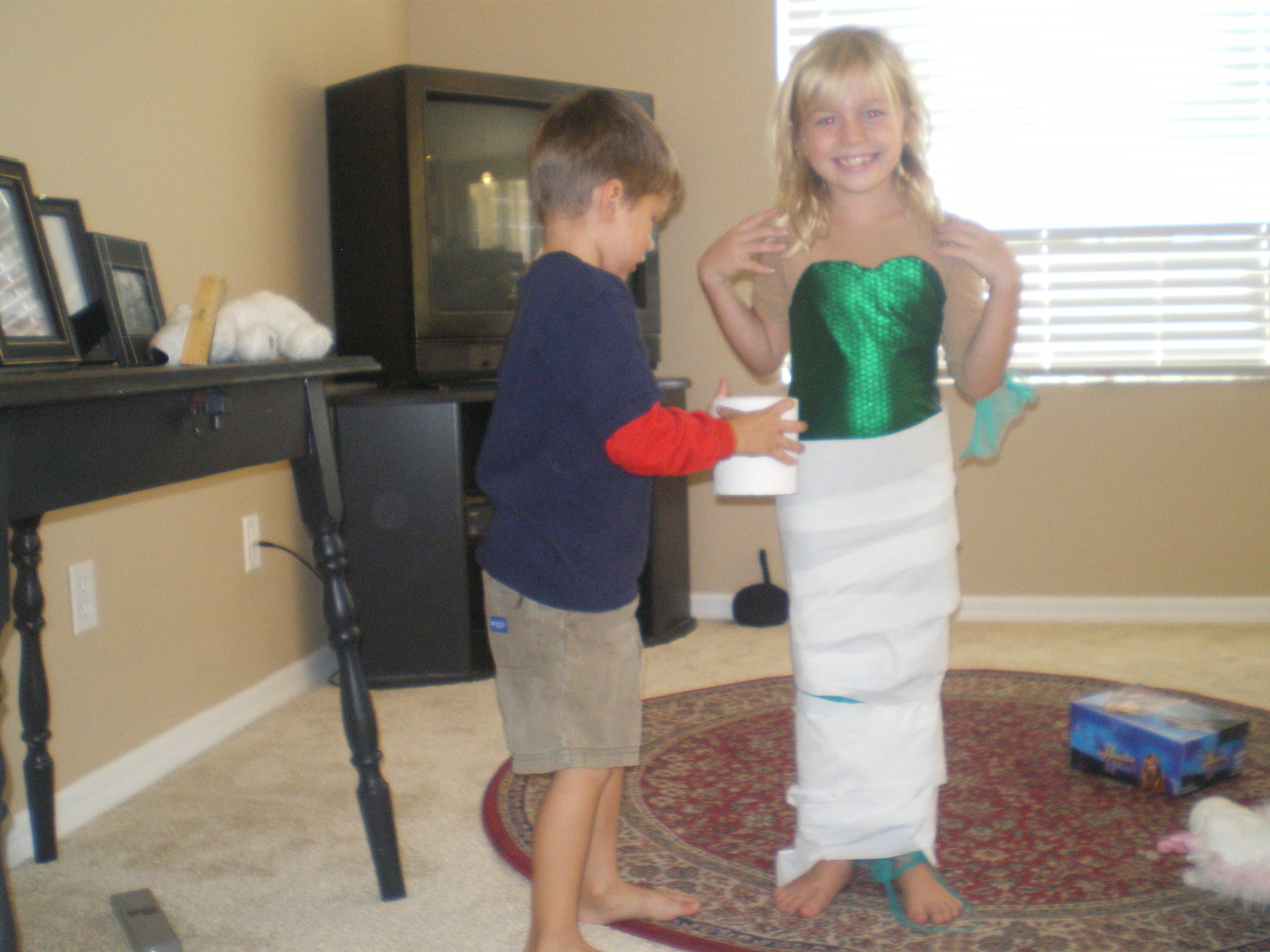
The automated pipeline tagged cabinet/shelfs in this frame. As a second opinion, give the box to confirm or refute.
[303,378,703,697]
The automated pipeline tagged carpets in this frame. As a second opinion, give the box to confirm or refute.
[477,665,1270,952]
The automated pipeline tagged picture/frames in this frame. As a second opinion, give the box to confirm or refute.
[31,193,92,318]
[86,228,169,369]
[0,154,84,370]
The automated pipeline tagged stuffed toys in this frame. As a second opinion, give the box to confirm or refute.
[149,291,334,362]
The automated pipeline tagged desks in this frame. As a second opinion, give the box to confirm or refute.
[0,349,410,952]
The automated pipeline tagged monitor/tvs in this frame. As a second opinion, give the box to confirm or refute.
[322,63,666,390]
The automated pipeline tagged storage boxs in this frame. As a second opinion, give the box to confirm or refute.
[1065,685,1251,798]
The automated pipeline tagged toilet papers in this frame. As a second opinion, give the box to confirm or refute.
[713,398,800,498]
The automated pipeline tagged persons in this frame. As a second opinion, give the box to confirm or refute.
[696,28,1019,926]
[476,89,807,952]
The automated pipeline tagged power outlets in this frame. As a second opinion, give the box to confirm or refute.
[66,558,100,636]
[240,512,265,577]
